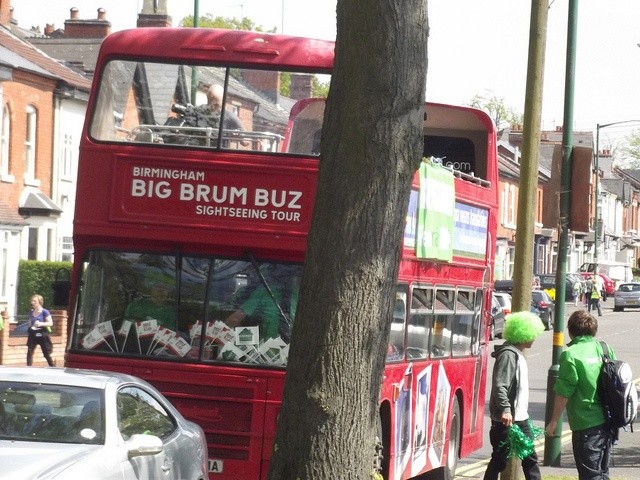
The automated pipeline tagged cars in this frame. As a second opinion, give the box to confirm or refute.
[490,295,505,340]
[0,367,209,480]
[532,291,556,330]
[614,283,640,311]
[566,272,586,300]
[494,293,512,315]
[579,272,615,297]
[534,275,574,301]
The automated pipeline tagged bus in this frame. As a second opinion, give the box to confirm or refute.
[52,27,500,480]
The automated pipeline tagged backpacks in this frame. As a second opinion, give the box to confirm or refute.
[600,341,638,440]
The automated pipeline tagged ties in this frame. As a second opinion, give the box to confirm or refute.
[280,276,292,345]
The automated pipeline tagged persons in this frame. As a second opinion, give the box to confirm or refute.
[181,84,245,150]
[26,295,56,366]
[539,311,618,480]
[572,278,606,316]
[483,311,545,480]
[122,282,176,331]
[222,281,300,346]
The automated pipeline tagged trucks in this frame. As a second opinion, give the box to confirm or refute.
[576,260,633,290]
